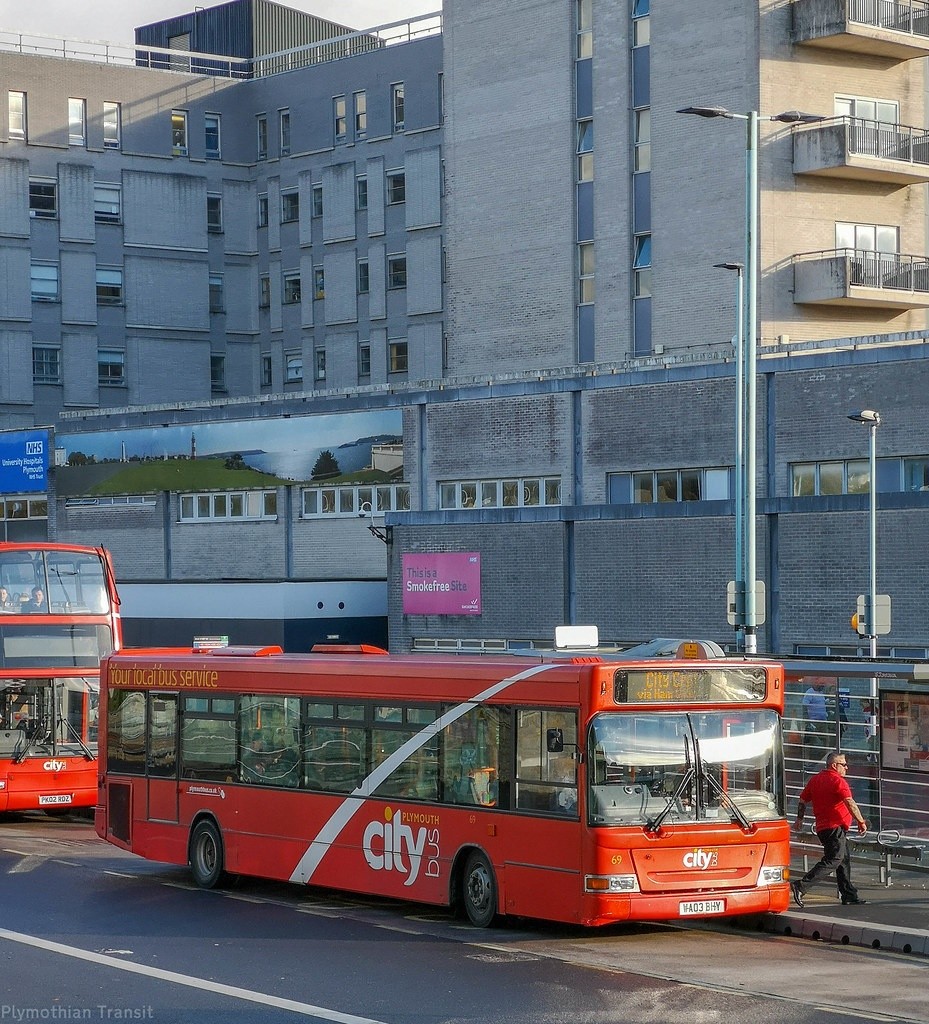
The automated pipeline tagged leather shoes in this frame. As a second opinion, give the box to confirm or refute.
[790,880,805,908]
[841,896,871,905]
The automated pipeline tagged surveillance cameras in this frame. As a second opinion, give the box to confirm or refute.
[358,510,366,517]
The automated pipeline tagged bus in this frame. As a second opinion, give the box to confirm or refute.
[90,641,794,928]
[0,537,125,821]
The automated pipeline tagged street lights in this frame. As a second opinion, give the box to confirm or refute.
[712,261,747,659]
[844,407,880,840]
[677,103,826,662]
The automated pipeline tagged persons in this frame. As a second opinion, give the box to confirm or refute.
[244,733,276,783]
[0,586,48,615]
[802,677,849,758]
[859,698,878,762]
[790,752,868,909]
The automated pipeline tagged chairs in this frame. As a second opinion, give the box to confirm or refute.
[8,593,90,613]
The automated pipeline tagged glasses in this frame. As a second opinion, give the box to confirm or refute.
[836,762,849,767]
